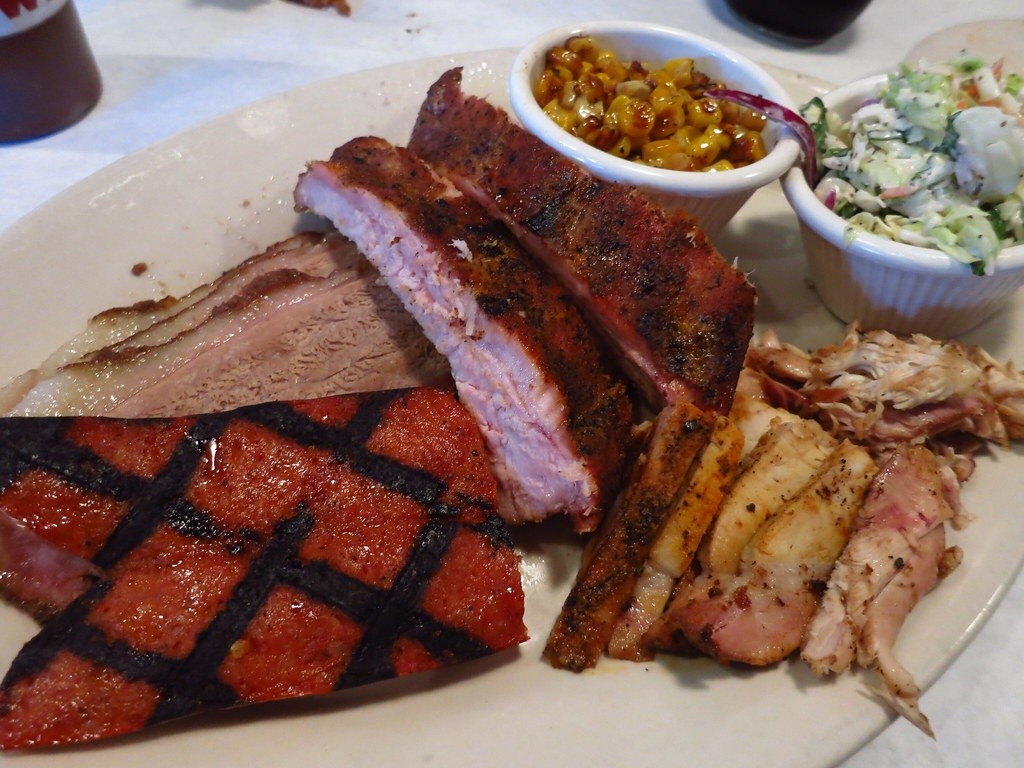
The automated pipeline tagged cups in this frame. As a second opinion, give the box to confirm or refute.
[781,73,1024,340]
[506,23,801,239]
[1,0,103,143]
[730,0,872,44]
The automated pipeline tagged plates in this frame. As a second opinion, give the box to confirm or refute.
[0,48,1024,768]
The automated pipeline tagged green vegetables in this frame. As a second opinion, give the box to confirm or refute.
[801,52,1024,278]
